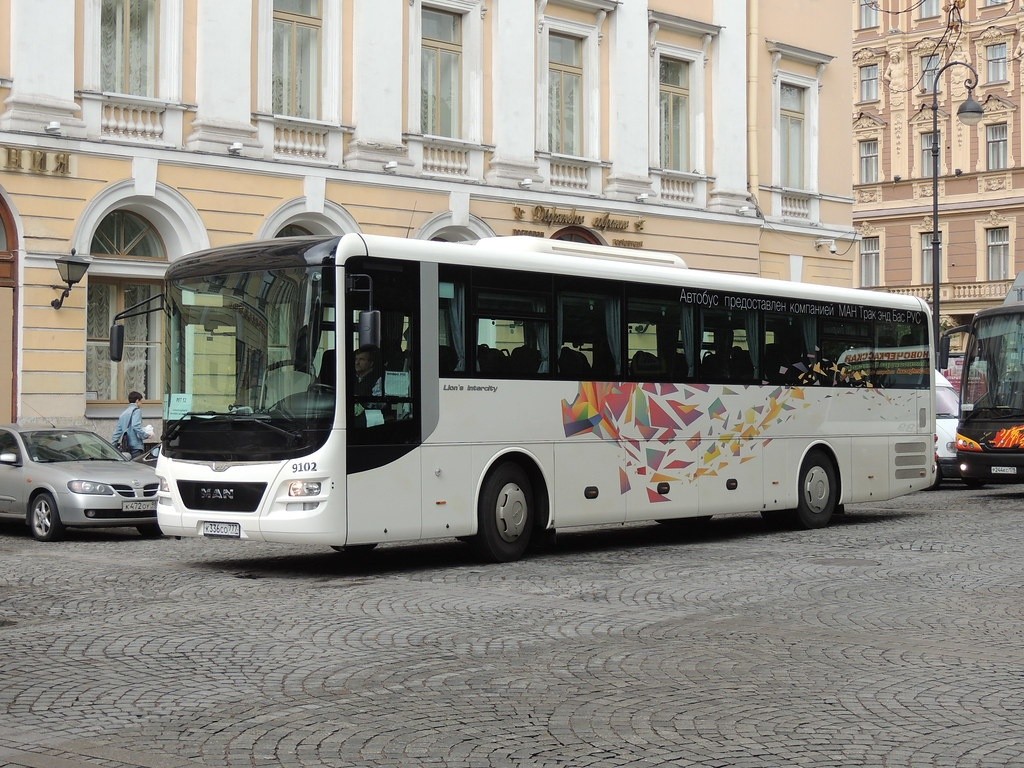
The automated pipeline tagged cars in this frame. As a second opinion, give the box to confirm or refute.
[0,423,160,542]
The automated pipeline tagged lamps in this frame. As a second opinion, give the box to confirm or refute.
[518,179,533,189]
[44,121,61,133]
[51,248,93,309]
[893,175,901,182]
[737,206,749,213]
[955,169,963,176]
[382,161,397,173]
[228,142,243,154]
[636,193,648,200]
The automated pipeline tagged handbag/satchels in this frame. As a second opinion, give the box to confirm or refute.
[137,425,154,439]
[117,428,128,453]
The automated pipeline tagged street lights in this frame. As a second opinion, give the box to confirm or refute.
[931,60,986,373]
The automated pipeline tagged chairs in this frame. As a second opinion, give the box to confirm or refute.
[260,344,869,407]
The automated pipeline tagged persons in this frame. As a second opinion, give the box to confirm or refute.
[353,347,383,397]
[112,391,147,459]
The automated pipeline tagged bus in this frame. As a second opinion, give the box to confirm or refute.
[112,233,941,566]
[938,306,1024,487]
[918,368,986,490]
